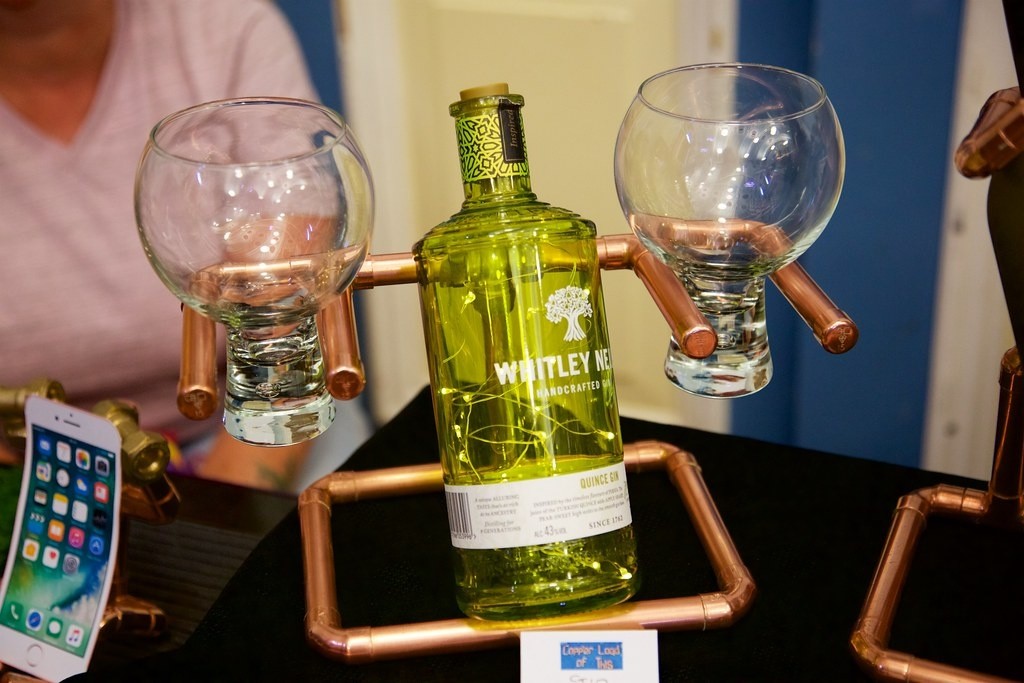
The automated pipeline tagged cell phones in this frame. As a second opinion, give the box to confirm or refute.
[1,396,122,683]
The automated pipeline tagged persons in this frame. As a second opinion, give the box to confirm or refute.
[0,0,373,495]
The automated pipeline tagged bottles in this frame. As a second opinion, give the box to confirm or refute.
[412,93,638,627]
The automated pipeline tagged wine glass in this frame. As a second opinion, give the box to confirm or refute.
[613,62,844,399]
[136,99,372,446]
